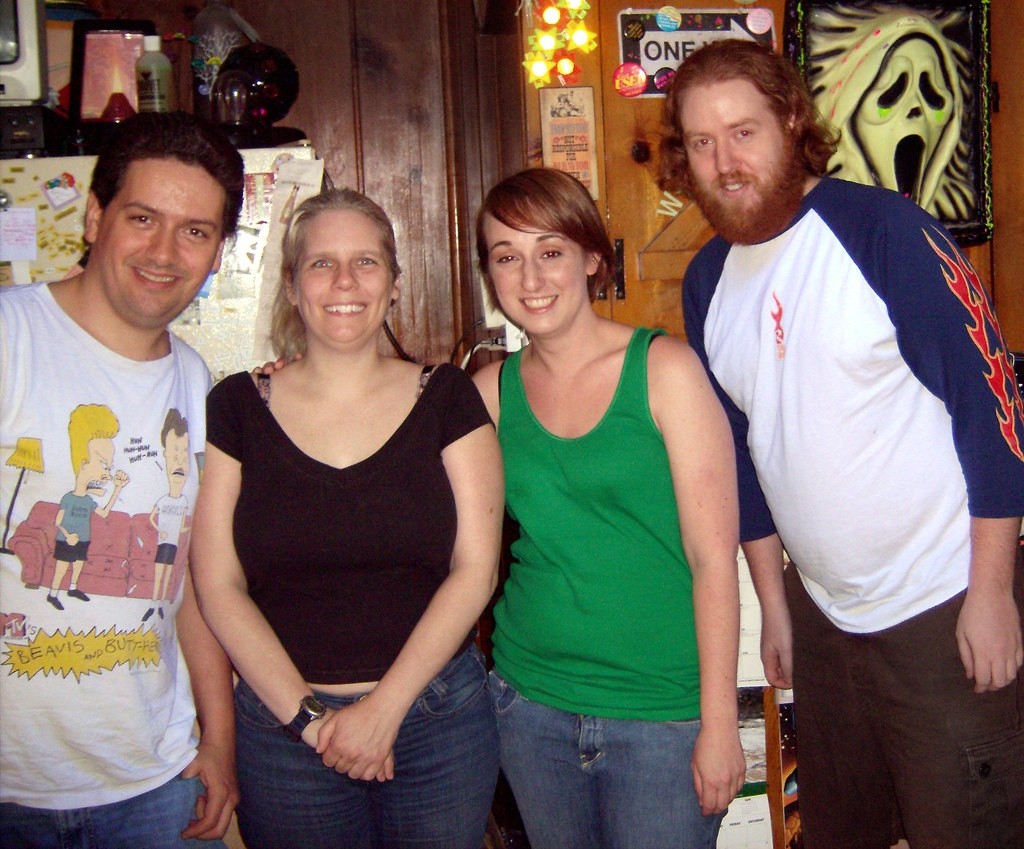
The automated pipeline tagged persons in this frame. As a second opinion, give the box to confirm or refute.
[660,40,1024,849]
[0,111,241,849]
[252,168,746,849]
[191,187,506,849]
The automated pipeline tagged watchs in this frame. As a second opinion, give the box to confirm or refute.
[287,696,324,738]
